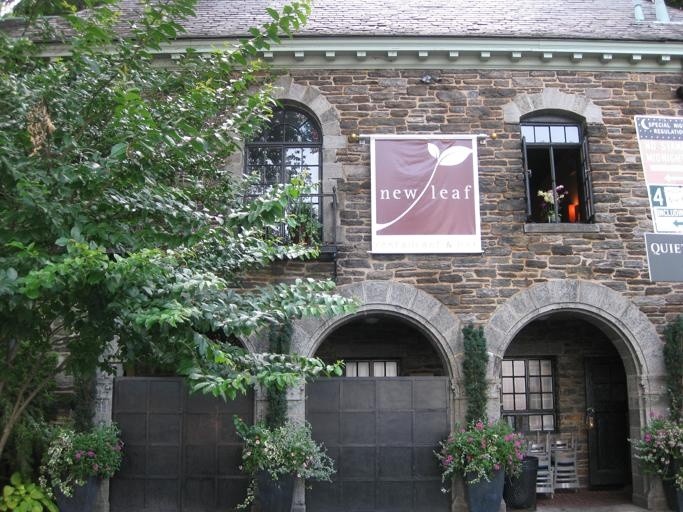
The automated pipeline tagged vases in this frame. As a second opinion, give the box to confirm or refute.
[463,466,507,512]
[505,455,537,510]
[255,476,310,510]
[55,470,112,511]
[661,472,682,511]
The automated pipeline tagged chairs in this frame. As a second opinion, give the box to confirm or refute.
[536,430,584,499]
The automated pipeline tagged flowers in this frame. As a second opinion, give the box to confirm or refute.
[43,422,127,470]
[438,419,525,475]
[236,419,338,479]
[632,407,682,467]
